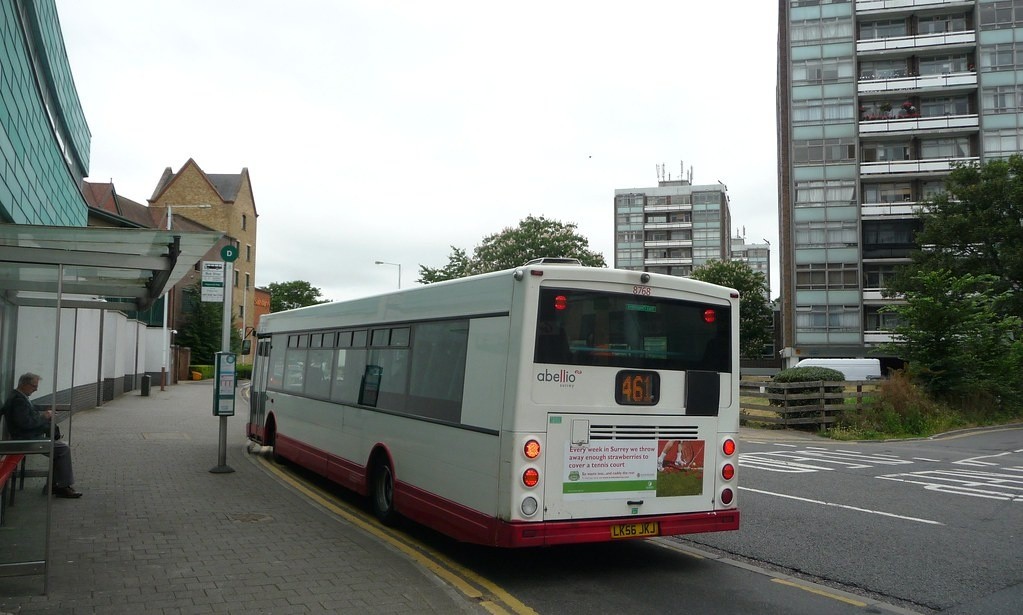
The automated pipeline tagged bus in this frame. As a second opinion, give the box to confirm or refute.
[241,257,740,548]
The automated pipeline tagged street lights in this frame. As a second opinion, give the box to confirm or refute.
[160,204,211,392]
[375,261,401,291]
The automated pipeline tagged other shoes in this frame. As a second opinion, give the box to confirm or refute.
[54,484,79,498]
[42,483,82,498]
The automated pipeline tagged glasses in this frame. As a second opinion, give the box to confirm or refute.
[26,382,38,388]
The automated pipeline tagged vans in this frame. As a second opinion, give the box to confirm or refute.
[793,359,881,380]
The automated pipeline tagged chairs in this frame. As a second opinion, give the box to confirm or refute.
[302,366,324,394]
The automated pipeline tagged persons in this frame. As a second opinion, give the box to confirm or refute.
[4,373,83,499]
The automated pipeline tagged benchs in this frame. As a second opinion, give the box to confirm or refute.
[0,451,26,528]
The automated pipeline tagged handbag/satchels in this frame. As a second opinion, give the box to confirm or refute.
[45,417,60,441]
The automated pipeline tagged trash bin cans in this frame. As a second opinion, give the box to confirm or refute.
[141,375,152,396]
